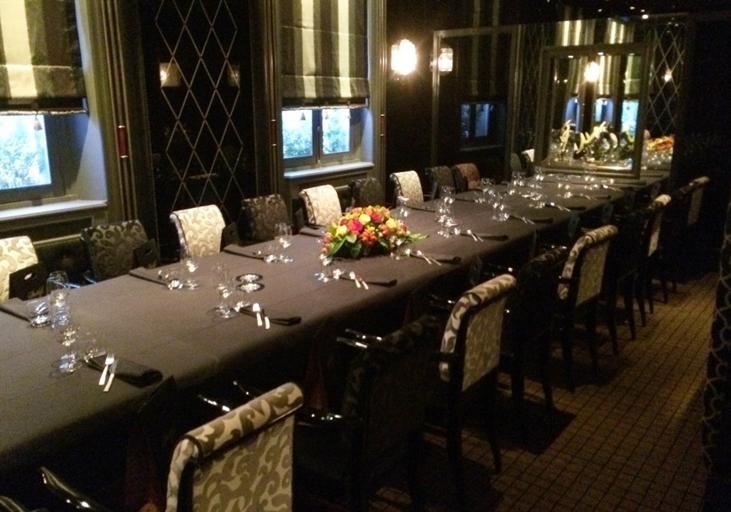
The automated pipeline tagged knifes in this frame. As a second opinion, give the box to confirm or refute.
[348,270,360,289]
[260,308,270,330]
[424,252,442,267]
[102,360,119,393]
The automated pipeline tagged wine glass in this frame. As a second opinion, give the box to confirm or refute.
[474,175,512,227]
[434,183,460,241]
[183,249,199,289]
[533,164,612,199]
[272,221,294,264]
[45,270,70,325]
[47,307,83,375]
[510,170,550,210]
[394,197,412,226]
[216,282,236,319]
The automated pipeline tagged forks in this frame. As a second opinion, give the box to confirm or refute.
[355,273,369,290]
[411,249,431,265]
[251,301,262,327]
[544,200,571,212]
[508,210,538,226]
[95,350,114,386]
[462,229,484,245]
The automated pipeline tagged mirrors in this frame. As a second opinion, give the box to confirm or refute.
[429,23,525,185]
[532,39,655,183]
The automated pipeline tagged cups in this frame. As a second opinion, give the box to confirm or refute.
[165,268,183,291]
[25,297,50,328]
[261,243,277,263]
